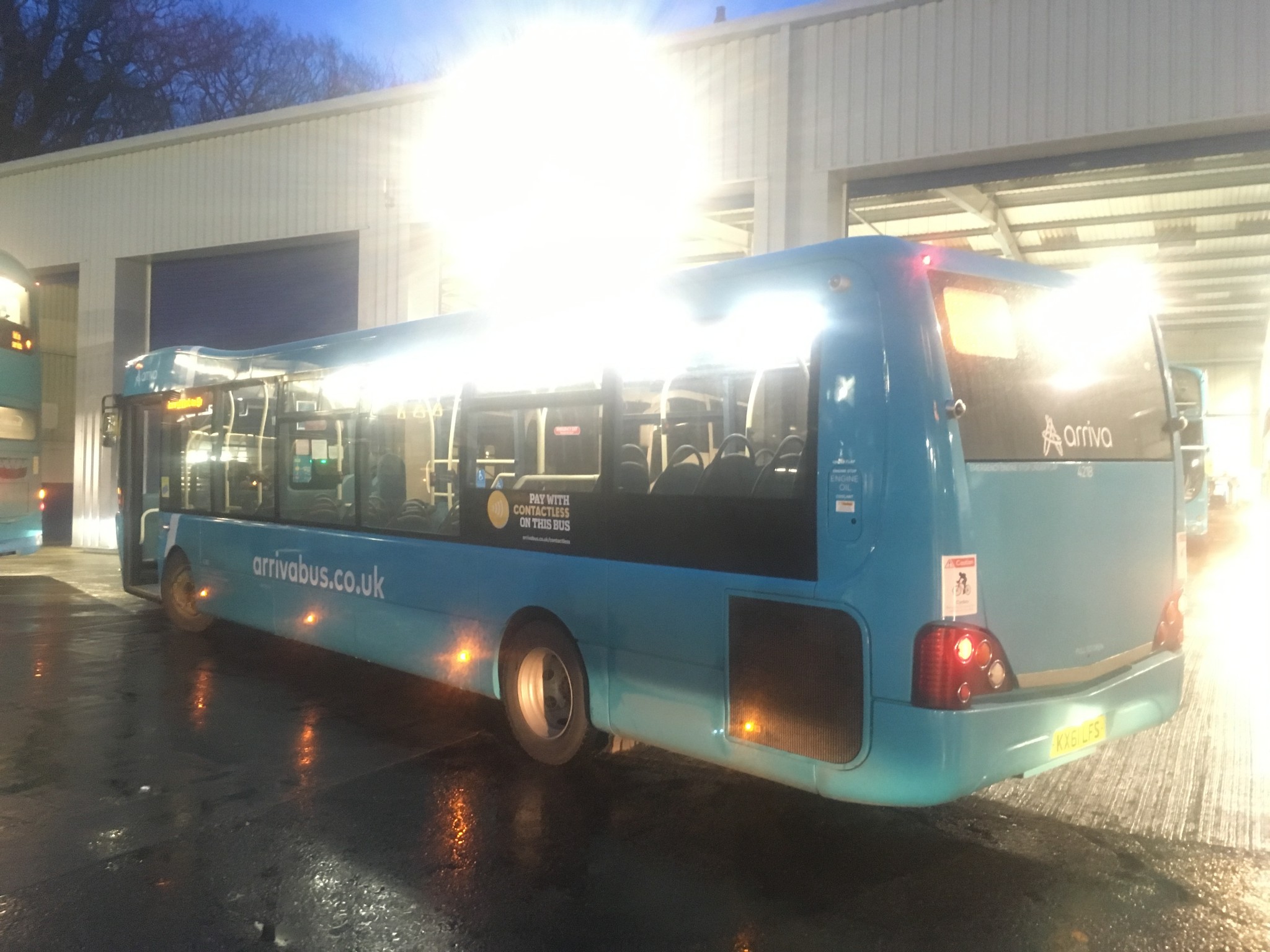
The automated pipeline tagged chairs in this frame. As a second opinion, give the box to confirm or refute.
[584,429,808,501]
[300,491,465,538]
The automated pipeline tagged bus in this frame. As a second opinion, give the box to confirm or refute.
[1164,363,1209,540]
[100,235,1191,813]
[0,249,47,559]
